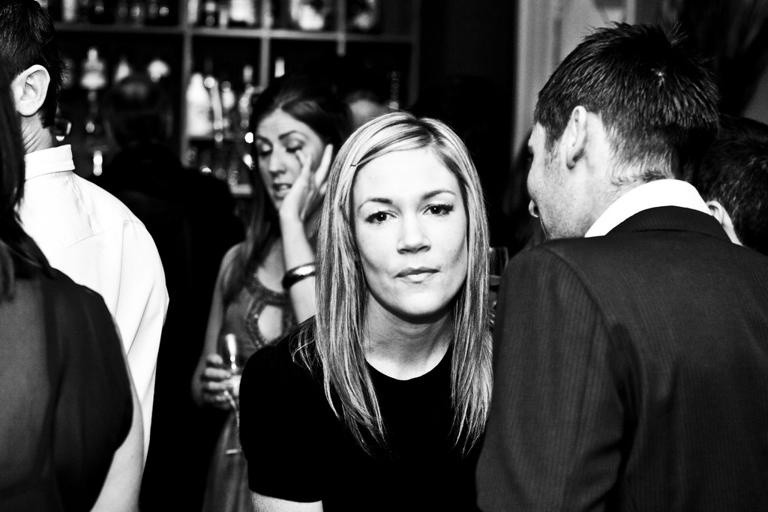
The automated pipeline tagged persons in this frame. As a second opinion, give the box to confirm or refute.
[234,109,495,511]
[89,73,248,512]
[472,19,768,512]
[0,53,146,511]
[2,0,174,512]
[190,68,357,512]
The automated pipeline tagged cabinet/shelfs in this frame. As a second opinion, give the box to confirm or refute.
[43,0,419,194]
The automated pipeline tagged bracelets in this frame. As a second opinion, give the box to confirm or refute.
[280,261,320,292]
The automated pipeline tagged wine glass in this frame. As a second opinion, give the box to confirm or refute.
[216,333,254,457]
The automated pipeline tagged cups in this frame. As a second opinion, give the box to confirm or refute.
[488,247,511,288]
